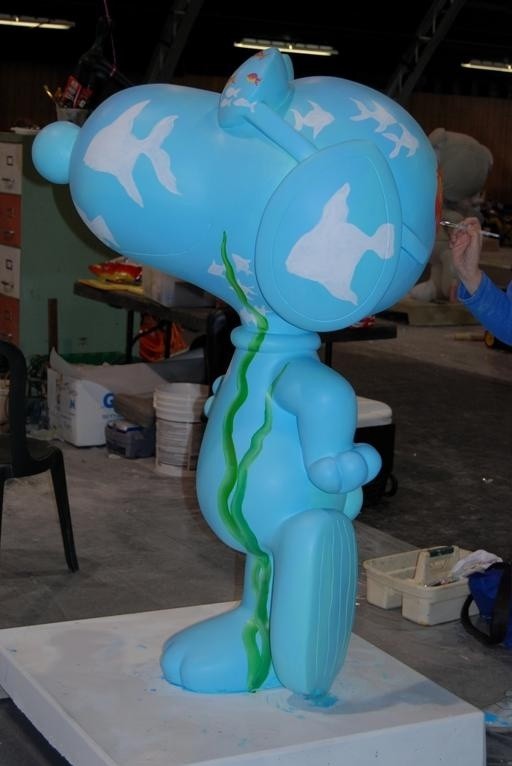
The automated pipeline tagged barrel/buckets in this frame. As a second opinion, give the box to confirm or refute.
[151,383,209,477]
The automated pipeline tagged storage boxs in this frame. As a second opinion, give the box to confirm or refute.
[46,362,169,448]
[105,420,155,459]
[363,544,480,627]
[140,266,226,310]
[354,395,398,508]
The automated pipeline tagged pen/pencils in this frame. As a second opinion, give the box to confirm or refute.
[439,220,500,239]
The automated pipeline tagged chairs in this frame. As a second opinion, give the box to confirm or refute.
[0,338,79,573]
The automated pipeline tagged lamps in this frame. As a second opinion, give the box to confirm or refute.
[0,14,75,31]
[461,59,512,73]
[232,39,338,56]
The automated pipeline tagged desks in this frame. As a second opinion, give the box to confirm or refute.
[73,279,398,424]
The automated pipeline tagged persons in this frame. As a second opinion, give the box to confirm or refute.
[446,214,512,353]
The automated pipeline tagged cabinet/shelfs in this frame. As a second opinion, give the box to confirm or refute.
[0,131,142,397]
